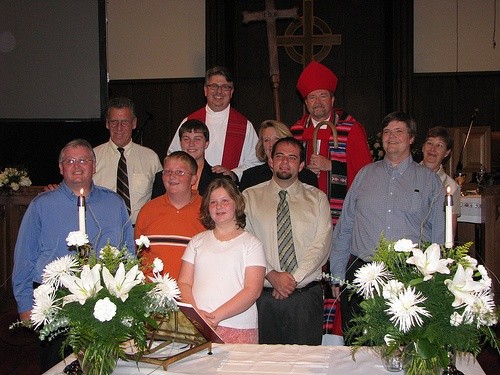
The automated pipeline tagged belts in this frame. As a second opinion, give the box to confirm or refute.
[263,282,319,292]
[131,224,135,228]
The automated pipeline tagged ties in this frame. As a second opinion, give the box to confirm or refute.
[276,190,298,274]
[117,147,132,217]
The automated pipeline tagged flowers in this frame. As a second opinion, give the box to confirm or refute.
[368,132,384,161]
[0,168,33,192]
[316,231,500,375]
[9,230,182,375]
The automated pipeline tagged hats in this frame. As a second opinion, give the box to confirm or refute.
[297,59,337,98]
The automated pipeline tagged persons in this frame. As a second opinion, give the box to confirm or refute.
[239,120,319,194]
[418,126,461,249]
[177,175,266,344]
[167,67,266,189]
[242,137,333,346]
[43,96,164,235]
[133,151,208,287]
[151,119,235,200]
[290,60,374,337]
[329,112,445,347]
[12,139,135,375]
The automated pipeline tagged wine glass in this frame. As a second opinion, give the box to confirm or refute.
[476,172,488,195]
[454,172,467,197]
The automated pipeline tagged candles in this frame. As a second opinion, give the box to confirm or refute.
[444,186,454,248]
[77,188,86,233]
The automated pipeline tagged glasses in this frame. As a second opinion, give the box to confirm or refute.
[109,118,135,128]
[65,158,94,164]
[164,170,192,177]
[207,83,232,92]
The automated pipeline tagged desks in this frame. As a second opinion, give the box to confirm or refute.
[44,342,488,375]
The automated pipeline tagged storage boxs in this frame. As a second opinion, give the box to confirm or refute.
[457,196,495,223]
